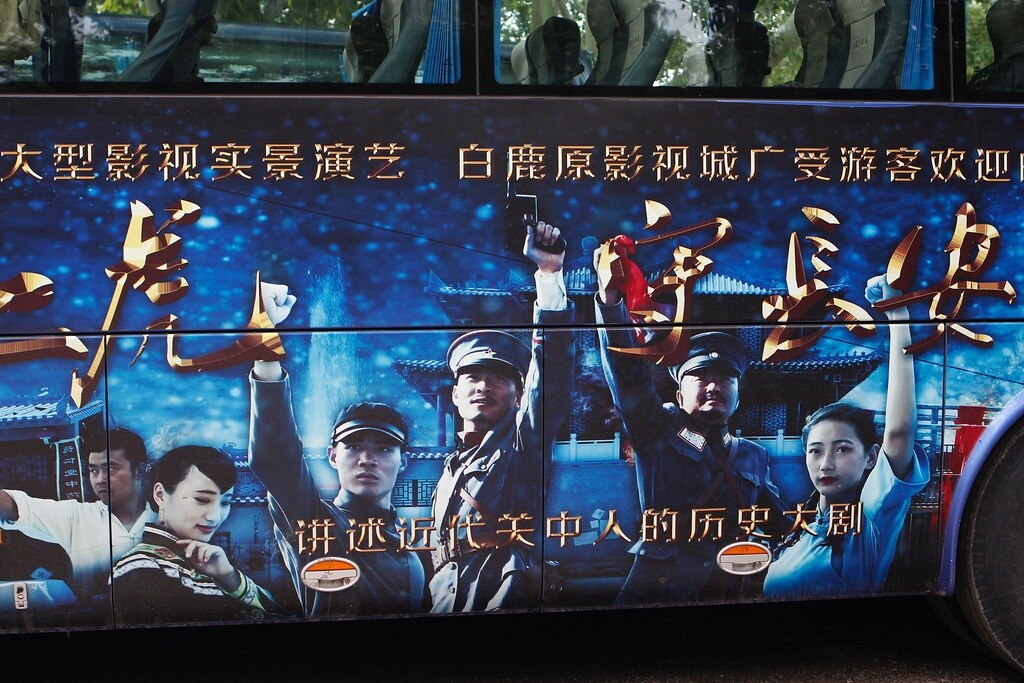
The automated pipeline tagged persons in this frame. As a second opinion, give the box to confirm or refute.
[763,273,930,602]
[429,214,576,614]
[0,428,157,606]
[108,445,277,624]
[248,281,434,621]
[594,243,798,606]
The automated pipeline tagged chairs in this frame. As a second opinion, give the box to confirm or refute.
[343,0,434,83]
[704,0,1024,94]
[26,0,216,83]
[512,0,671,86]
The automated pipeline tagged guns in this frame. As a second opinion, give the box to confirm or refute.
[505,150,567,255]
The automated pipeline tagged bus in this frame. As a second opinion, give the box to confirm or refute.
[0,0,1024,677]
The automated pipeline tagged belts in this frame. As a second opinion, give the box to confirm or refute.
[431,533,513,571]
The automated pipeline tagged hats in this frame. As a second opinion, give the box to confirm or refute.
[446,329,532,391]
[668,332,749,383]
[331,402,408,444]
